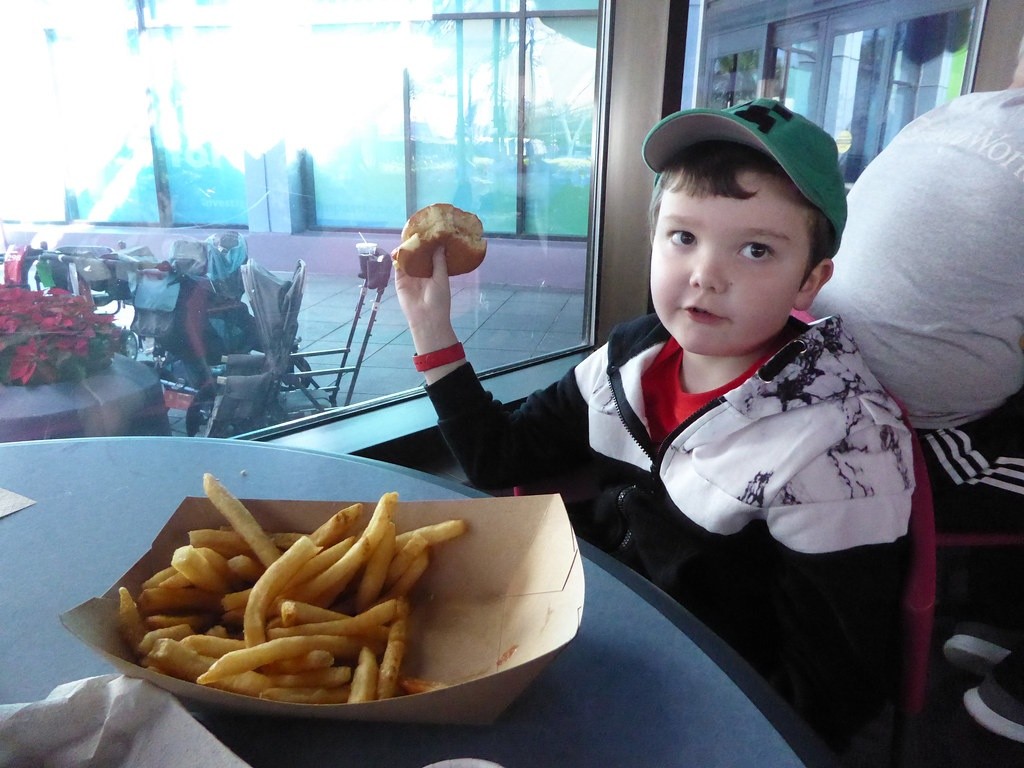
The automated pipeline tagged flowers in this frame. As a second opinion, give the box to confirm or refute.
[0,278,121,388]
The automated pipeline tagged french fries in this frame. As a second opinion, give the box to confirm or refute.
[119,472,467,705]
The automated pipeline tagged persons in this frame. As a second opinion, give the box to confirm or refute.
[802,34,1024,742]
[387,98,916,768]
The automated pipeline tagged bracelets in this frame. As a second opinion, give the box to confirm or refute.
[412,341,466,372]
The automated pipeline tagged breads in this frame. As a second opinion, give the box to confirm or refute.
[391,204,486,277]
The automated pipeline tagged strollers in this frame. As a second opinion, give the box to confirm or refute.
[0,228,392,437]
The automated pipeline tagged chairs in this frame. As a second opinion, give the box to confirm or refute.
[512,308,1024,768]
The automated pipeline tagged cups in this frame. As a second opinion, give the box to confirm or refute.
[356,243,377,257]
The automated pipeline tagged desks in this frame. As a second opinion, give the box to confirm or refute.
[0,352,173,442]
[0,436,844,768]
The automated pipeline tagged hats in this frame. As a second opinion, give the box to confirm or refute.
[643,98,848,255]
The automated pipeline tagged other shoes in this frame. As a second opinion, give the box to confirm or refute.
[964,674,1024,747]
[941,623,1012,671]
